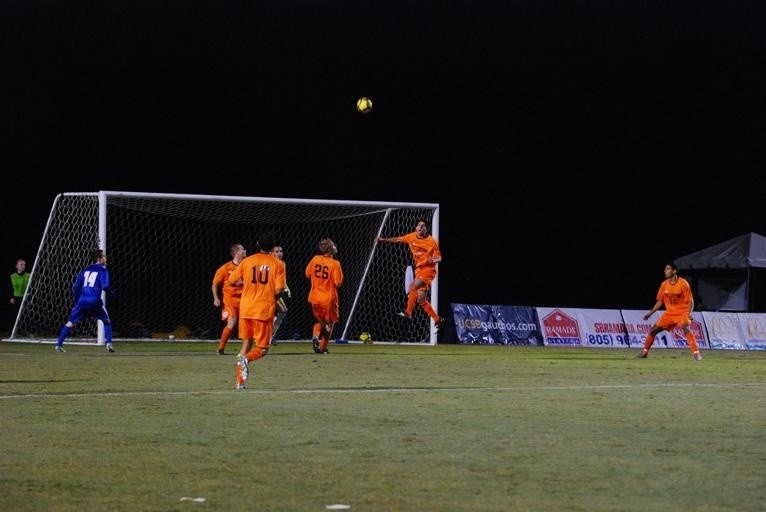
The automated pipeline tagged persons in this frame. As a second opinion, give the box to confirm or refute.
[268,244,293,345]
[6,258,34,339]
[54,249,114,352]
[374,220,446,335]
[305,239,345,353]
[211,243,248,355]
[228,234,287,389]
[635,262,702,361]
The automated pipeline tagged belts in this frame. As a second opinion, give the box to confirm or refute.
[311,337,322,353]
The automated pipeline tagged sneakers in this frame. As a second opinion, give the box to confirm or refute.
[56,346,65,353]
[236,355,249,390]
[106,344,115,352]
[433,318,445,335]
[397,312,412,319]
[693,352,702,363]
[636,352,647,358]
[217,349,225,355]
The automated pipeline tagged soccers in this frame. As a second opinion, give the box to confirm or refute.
[359,332,371,341]
[356,97,372,112]
